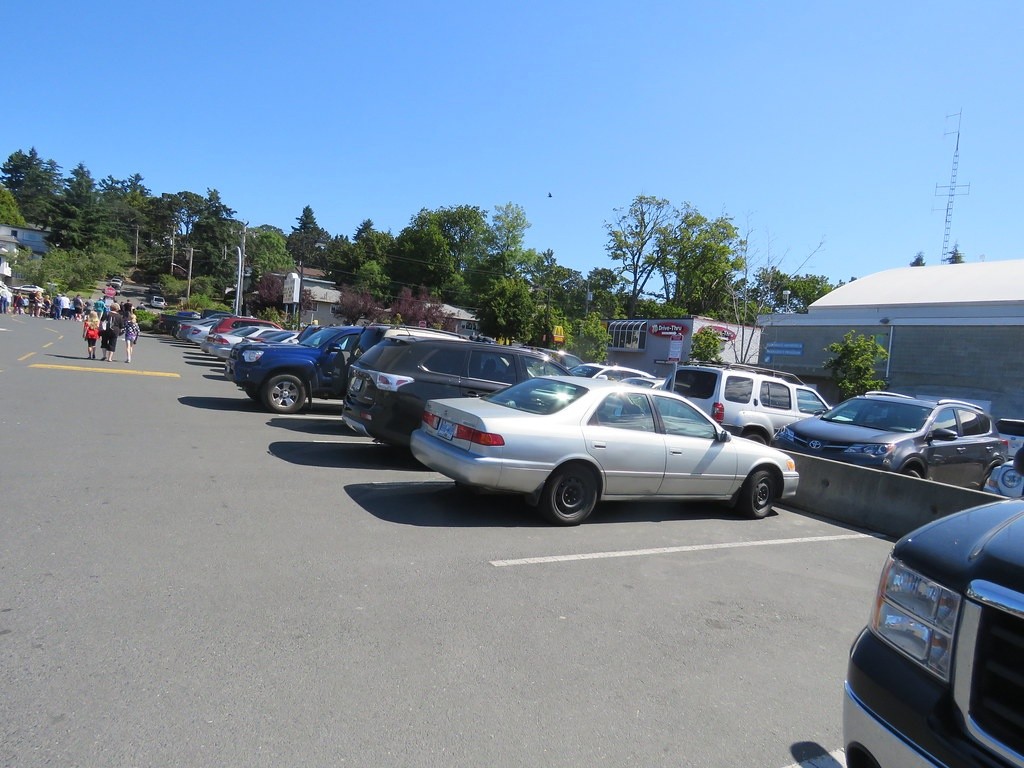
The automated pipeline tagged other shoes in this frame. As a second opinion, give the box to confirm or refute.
[107,359,113,362]
[101,357,107,361]
[93,355,95,358]
[87,356,91,359]
[125,358,132,363]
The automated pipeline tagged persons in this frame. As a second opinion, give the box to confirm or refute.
[0,284,139,362]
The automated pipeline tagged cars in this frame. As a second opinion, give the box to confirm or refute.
[411,376,800,526]
[523,345,584,369]
[107,275,123,296]
[209,326,282,359]
[187,322,218,343]
[570,362,655,383]
[12,284,47,296]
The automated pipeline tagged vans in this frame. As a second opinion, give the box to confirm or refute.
[660,359,831,448]
[150,296,165,309]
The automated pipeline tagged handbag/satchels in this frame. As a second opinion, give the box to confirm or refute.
[99,311,109,333]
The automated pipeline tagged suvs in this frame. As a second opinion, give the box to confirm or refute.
[769,391,1010,491]
[222,326,363,415]
[210,317,284,334]
[340,321,575,446]
[994,417,1024,461]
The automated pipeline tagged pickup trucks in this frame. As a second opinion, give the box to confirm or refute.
[159,309,238,340]
